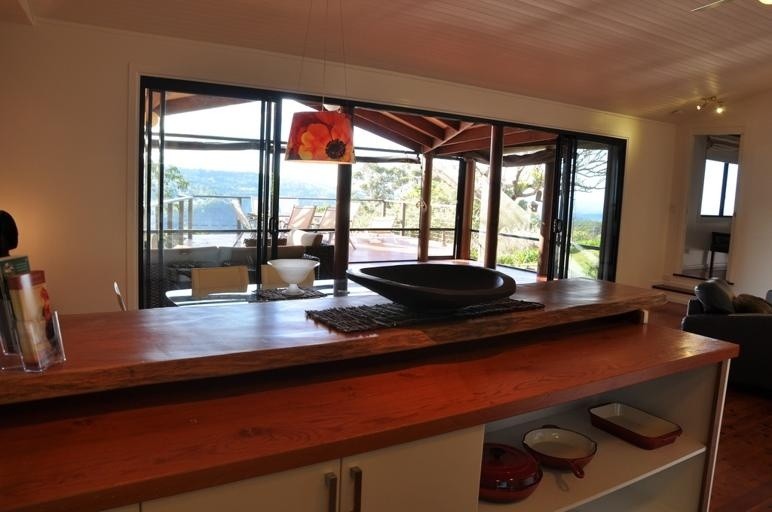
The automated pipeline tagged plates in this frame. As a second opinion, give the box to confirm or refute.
[345,264,517,310]
[588,399,683,450]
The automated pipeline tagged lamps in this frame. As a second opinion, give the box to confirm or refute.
[697,96,725,114]
[284,0,358,165]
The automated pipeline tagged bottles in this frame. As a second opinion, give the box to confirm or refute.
[6,268,56,364]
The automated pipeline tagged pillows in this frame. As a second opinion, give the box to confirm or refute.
[732,287,772,316]
[695,276,736,314]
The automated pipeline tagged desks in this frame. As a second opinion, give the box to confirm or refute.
[709,231,730,278]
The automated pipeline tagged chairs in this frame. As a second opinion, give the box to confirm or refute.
[112,280,126,312]
[260,264,314,294]
[678,296,772,401]
[191,261,251,302]
[229,196,357,252]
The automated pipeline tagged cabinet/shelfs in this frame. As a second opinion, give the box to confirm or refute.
[2,325,742,510]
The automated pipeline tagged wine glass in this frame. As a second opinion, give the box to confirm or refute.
[267,258,321,296]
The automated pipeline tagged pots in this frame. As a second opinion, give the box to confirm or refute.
[478,442,544,505]
[521,423,598,480]
